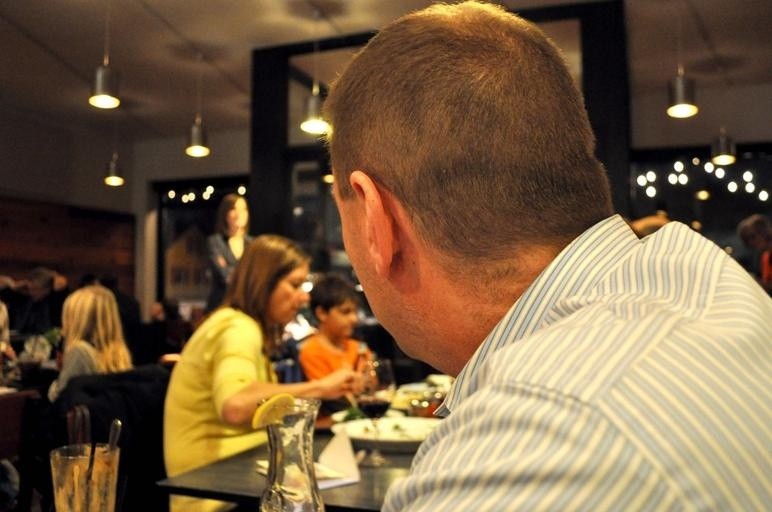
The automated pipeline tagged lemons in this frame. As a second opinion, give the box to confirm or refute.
[251,393,297,430]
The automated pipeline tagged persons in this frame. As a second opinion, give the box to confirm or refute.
[0,267,194,404]
[737,214,772,296]
[163,195,377,512]
[323,0,772,511]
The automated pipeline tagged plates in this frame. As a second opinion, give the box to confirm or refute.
[328,373,455,459]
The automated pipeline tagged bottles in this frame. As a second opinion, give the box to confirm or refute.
[256,397,326,511]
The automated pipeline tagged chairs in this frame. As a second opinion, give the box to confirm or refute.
[1,389,43,512]
[22,365,171,512]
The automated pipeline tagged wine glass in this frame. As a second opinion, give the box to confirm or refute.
[354,355,397,468]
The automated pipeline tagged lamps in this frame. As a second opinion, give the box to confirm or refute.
[87,1,123,108]
[712,108,735,166]
[103,130,124,187]
[300,6,334,135]
[185,80,211,158]
[666,14,700,118]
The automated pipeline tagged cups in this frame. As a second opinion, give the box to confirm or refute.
[48,442,123,511]
